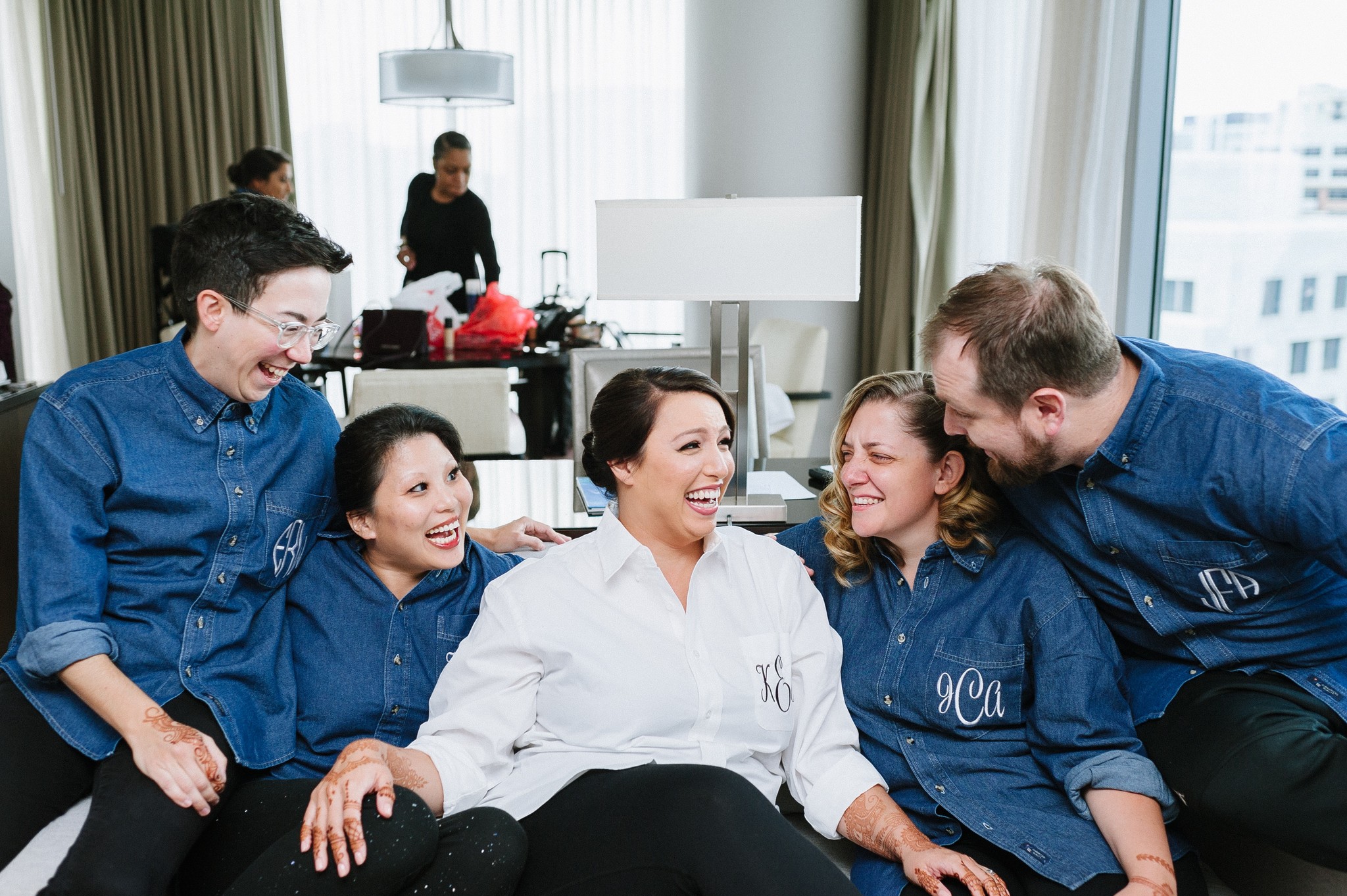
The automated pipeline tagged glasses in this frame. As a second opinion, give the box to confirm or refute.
[186,292,340,351]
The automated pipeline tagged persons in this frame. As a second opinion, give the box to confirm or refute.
[294,359,1013,896]
[225,141,293,202]
[918,251,1347,895]
[3,192,578,896]
[391,128,501,304]
[223,403,556,895]
[757,366,1179,895]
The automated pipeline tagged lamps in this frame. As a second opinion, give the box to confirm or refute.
[376,0,517,108]
[596,196,865,523]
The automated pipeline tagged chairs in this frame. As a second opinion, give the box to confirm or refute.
[747,320,833,467]
[343,366,529,462]
[567,343,771,464]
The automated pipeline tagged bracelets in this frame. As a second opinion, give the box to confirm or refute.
[398,241,408,249]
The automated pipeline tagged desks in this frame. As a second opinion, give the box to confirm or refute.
[308,323,635,460]
[454,456,839,542]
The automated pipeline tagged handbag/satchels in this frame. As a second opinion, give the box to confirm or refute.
[454,281,537,350]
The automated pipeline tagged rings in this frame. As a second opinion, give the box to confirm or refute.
[985,868,996,874]
